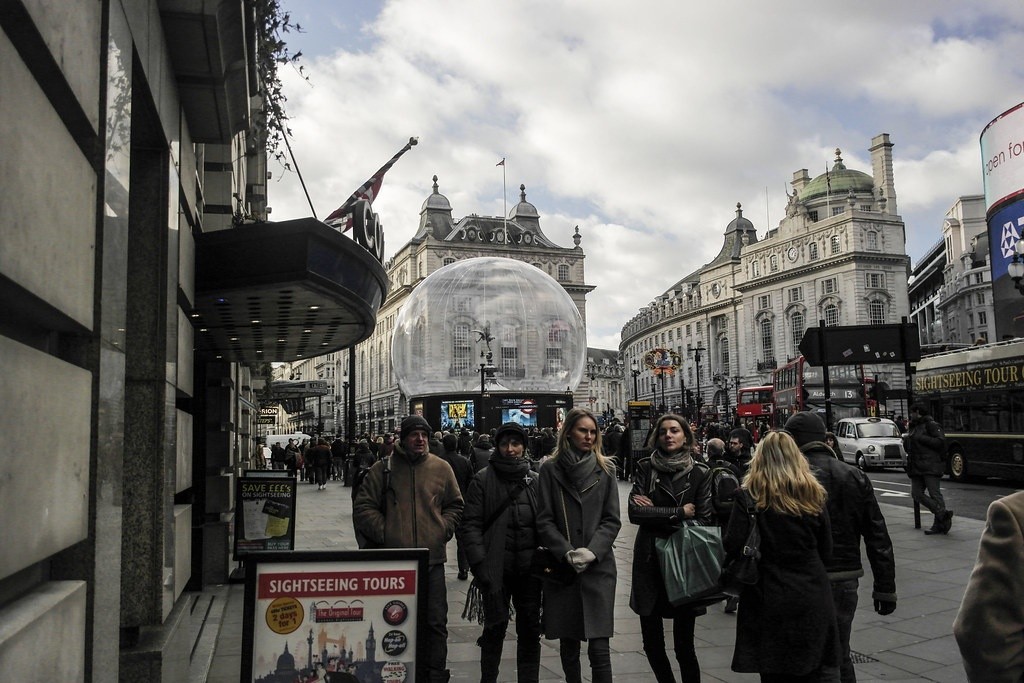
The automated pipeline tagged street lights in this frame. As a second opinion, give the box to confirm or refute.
[476,351,490,433]
[655,367,670,416]
[687,347,707,427]
[629,359,641,401]
[650,382,658,412]
[340,368,349,441]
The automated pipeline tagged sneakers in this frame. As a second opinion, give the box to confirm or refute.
[924,519,941,535]
[942,510,954,534]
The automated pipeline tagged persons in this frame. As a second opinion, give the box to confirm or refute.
[628,414,713,683]
[894,416,907,435]
[271,415,770,614]
[535,408,622,683]
[783,411,897,683]
[722,432,844,683]
[455,422,541,683]
[352,415,465,683]
[901,403,953,535]
[824,432,844,462]
[953,490,1024,683]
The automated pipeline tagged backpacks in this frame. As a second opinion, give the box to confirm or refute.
[926,420,949,461]
[706,459,739,517]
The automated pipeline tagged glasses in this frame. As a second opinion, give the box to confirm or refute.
[763,430,796,443]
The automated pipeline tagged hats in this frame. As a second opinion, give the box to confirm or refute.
[784,411,827,444]
[495,423,528,450]
[401,415,432,440]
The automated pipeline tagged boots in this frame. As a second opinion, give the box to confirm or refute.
[517,643,541,683]
[478,641,503,683]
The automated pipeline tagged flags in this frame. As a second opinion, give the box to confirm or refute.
[496,160,504,166]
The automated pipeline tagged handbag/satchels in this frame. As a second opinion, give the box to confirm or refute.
[655,518,726,608]
[729,485,762,586]
[534,549,574,586]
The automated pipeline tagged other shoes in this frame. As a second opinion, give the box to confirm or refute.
[323,485,326,489]
[725,597,739,613]
[318,486,322,489]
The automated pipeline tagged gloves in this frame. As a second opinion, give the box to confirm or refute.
[873,599,896,615]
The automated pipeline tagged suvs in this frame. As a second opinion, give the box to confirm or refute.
[834,416,909,473]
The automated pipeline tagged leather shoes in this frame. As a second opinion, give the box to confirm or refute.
[458,568,469,579]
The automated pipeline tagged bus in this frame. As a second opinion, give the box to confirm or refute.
[912,337,1024,488]
[735,384,774,443]
[772,355,877,435]
